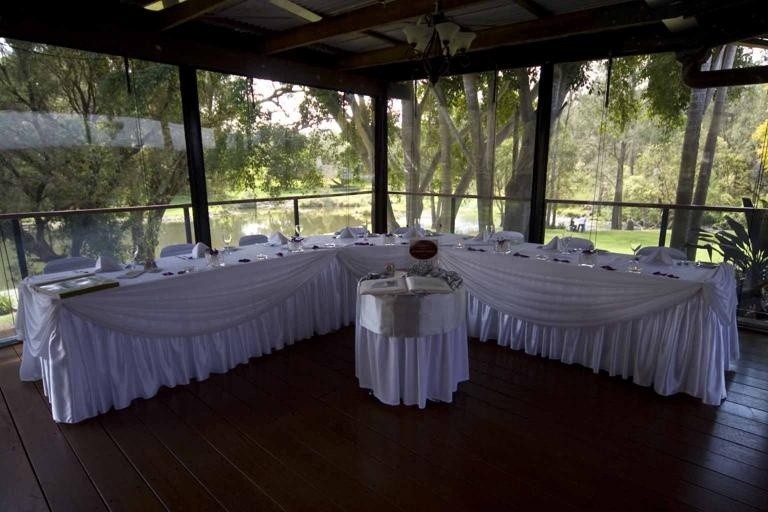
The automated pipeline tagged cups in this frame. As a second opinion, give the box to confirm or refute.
[454,224,642,273]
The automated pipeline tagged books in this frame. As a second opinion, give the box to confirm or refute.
[359,275,453,296]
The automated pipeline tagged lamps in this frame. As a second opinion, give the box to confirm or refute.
[396,0,478,64]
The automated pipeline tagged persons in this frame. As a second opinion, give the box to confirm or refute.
[578,214,585,232]
[569,217,577,231]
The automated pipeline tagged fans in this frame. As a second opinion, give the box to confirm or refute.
[142,0,323,25]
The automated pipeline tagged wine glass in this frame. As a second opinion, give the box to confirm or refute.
[128,217,419,278]
